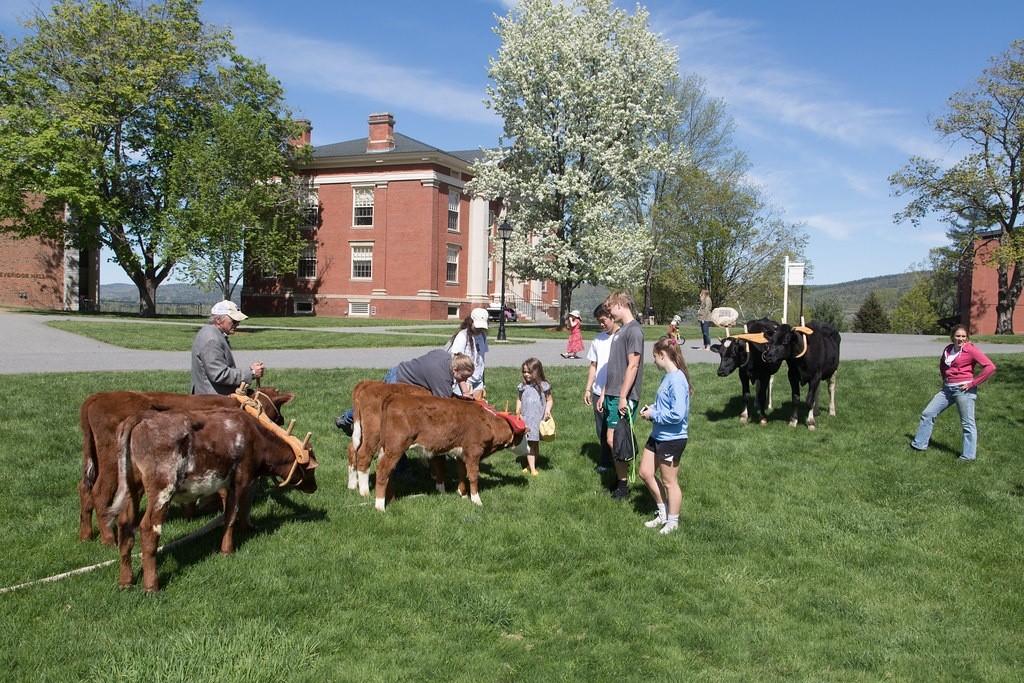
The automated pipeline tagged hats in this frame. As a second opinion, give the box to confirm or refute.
[211,300,249,322]
[471,308,489,329]
[569,310,582,322]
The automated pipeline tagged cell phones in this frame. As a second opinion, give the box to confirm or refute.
[640,406,655,423]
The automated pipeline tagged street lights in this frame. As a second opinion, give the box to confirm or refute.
[494,219,514,344]
[800,271,807,326]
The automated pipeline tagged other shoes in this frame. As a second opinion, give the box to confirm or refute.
[570,356,576,359]
[697,345,706,350]
[658,521,678,534]
[531,470,538,475]
[644,510,667,528]
[522,466,530,473]
[596,463,612,472]
[705,344,710,351]
[612,480,629,499]
[560,353,568,358]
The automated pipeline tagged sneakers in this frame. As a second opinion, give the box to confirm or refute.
[335,416,353,437]
[396,467,420,481]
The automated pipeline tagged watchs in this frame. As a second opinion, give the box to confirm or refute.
[252,370,255,374]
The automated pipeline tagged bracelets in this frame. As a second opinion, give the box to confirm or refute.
[462,392,469,394]
[585,389,591,392]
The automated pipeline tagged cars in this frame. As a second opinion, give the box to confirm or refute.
[487,303,520,323]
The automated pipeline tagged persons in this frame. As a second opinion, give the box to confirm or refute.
[189,300,264,519]
[911,324,997,460]
[335,348,475,477]
[452,307,489,398]
[668,321,677,342]
[516,358,554,474]
[583,292,644,500]
[697,290,713,347]
[638,338,693,535]
[561,310,585,358]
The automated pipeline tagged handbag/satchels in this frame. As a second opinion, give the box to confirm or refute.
[539,415,555,442]
[613,406,639,461]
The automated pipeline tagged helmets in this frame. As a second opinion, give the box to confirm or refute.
[673,315,681,321]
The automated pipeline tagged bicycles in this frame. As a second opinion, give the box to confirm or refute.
[659,327,686,345]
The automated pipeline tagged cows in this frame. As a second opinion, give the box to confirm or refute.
[349,379,527,510]
[78,386,320,595]
[709,318,782,425]
[761,320,843,430]
